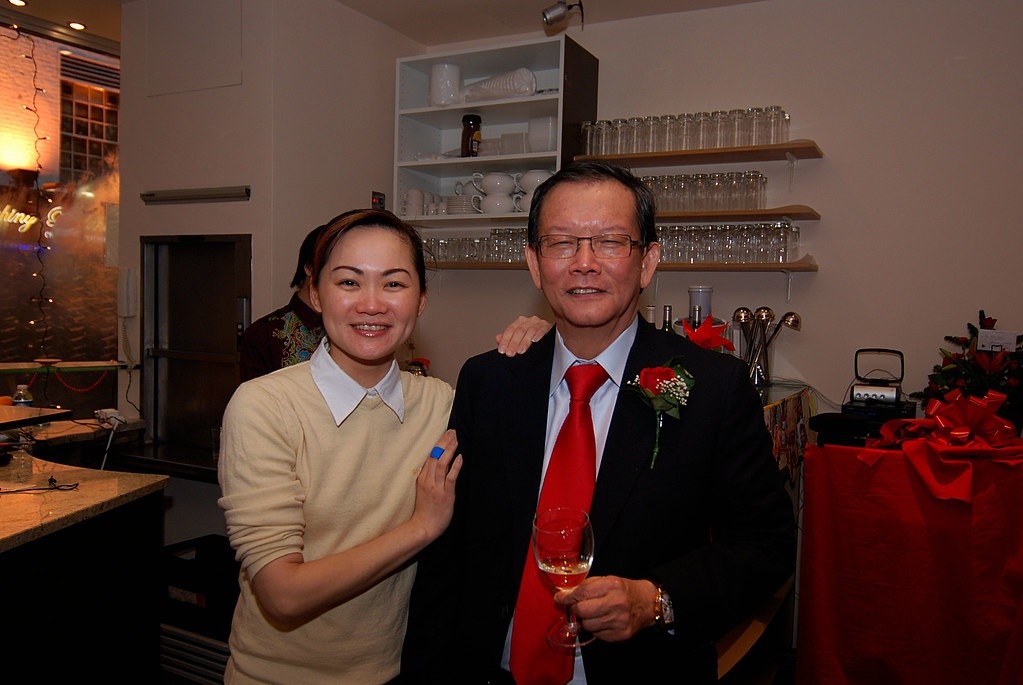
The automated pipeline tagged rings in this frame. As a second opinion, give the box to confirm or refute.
[430,448,444,458]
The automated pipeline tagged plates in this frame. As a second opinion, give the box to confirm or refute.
[448,195,480,214]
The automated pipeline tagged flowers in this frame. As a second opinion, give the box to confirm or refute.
[681,311,738,352]
[626,360,696,470]
[907,307,1023,410]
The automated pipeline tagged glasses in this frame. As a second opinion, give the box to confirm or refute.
[536,235,643,258]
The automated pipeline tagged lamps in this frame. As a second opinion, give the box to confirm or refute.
[540,0,586,25]
[7,169,37,188]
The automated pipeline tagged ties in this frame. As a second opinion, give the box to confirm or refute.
[509,364,609,685]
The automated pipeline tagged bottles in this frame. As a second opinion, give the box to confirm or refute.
[661,305,676,335]
[687,306,701,340]
[11,385,33,460]
[647,306,656,329]
[462,115,481,157]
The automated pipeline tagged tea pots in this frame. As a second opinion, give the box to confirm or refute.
[455,170,514,214]
[514,170,555,212]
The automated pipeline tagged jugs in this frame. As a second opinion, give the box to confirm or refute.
[403,361,429,378]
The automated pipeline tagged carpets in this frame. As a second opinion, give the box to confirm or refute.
[163,534,240,644]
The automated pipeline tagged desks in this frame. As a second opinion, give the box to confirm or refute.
[795,442,1023,685]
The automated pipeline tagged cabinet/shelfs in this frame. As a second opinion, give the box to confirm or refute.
[425,139,825,273]
[393,34,600,228]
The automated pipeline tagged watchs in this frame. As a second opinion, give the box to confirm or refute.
[654,585,674,625]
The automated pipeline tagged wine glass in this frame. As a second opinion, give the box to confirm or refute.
[533,507,596,647]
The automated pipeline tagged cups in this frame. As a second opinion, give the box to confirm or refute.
[430,64,460,106]
[581,115,679,155]
[422,238,489,263]
[728,323,782,387]
[489,228,527,262]
[400,189,447,215]
[464,68,536,102]
[638,171,768,213]
[678,106,790,151]
[655,223,801,263]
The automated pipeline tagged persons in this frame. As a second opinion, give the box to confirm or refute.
[214,211,554,685]
[397,155,799,685]
